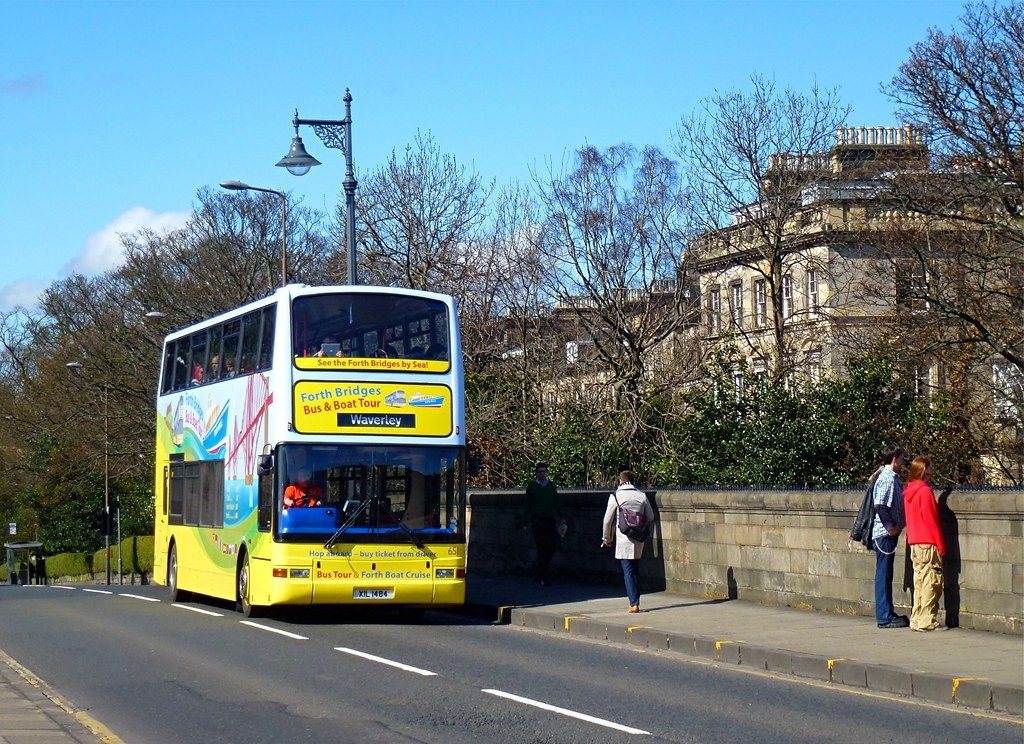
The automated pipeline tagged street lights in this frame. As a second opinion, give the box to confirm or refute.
[67,362,112,585]
[276,88,366,359]
[218,179,288,287]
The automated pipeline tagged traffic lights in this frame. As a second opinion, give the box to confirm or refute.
[95,507,108,537]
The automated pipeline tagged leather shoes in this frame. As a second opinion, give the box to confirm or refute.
[629,605,638,613]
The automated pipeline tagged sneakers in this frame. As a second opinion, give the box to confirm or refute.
[878,615,909,628]
[919,625,948,633]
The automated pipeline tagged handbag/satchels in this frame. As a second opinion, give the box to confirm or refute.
[619,508,649,541]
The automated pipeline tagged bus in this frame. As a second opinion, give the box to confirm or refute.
[154,284,478,617]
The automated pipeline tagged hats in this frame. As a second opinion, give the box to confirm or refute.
[227,360,235,367]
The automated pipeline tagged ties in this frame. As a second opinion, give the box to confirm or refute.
[301,487,309,507]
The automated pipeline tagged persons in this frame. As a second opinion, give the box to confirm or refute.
[188,333,447,388]
[600,471,653,614]
[283,466,325,509]
[522,463,567,588]
[10,556,47,586]
[868,444,909,628]
[902,455,948,632]
